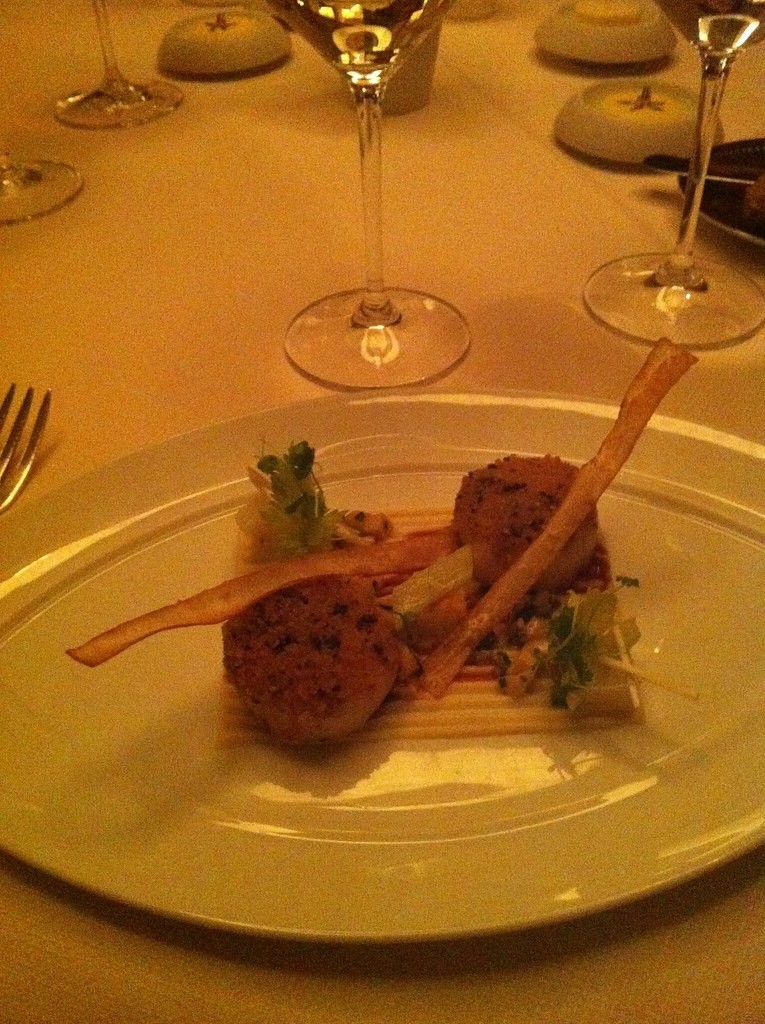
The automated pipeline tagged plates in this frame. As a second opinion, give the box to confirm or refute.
[0,390,765,942]
[678,139,765,251]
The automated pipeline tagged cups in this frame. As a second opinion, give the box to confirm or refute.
[378,24,441,114]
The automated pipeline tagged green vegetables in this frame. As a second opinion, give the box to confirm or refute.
[492,574,642,707]
[256,440,351,556]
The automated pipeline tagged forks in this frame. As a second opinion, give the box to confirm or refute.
[0,383,51,513]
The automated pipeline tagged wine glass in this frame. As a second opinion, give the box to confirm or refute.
[52,0,183,129]
[266,0,472,389]
[583,0,765,346]
[1,144,81,222]
